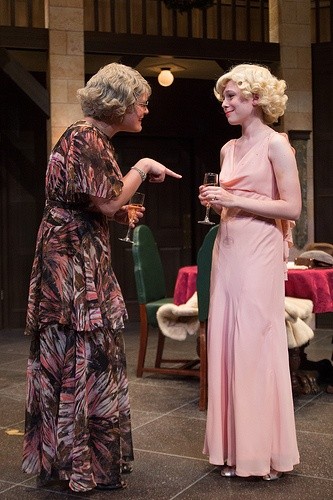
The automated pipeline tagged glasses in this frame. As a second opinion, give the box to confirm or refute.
[138,100,149,109]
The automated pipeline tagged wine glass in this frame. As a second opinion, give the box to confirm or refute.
[118,192,145,244]
[198,173,218,224]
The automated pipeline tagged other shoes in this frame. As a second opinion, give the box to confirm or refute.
[121,462,133,474]
[220,465,239,477]
[262,470,284,480]
[93,477,128,490]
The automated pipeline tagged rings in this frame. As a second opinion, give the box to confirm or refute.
[212,195,217,201]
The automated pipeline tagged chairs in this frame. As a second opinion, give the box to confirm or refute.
[196,224,236,412]
[132,225,203,382]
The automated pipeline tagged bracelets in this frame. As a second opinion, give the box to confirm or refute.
[131,166,146,182]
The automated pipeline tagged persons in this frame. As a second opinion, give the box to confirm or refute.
[22,62,184,494]
[197,63,302,481]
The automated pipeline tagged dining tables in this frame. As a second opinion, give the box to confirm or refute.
[173,262,333,383]
